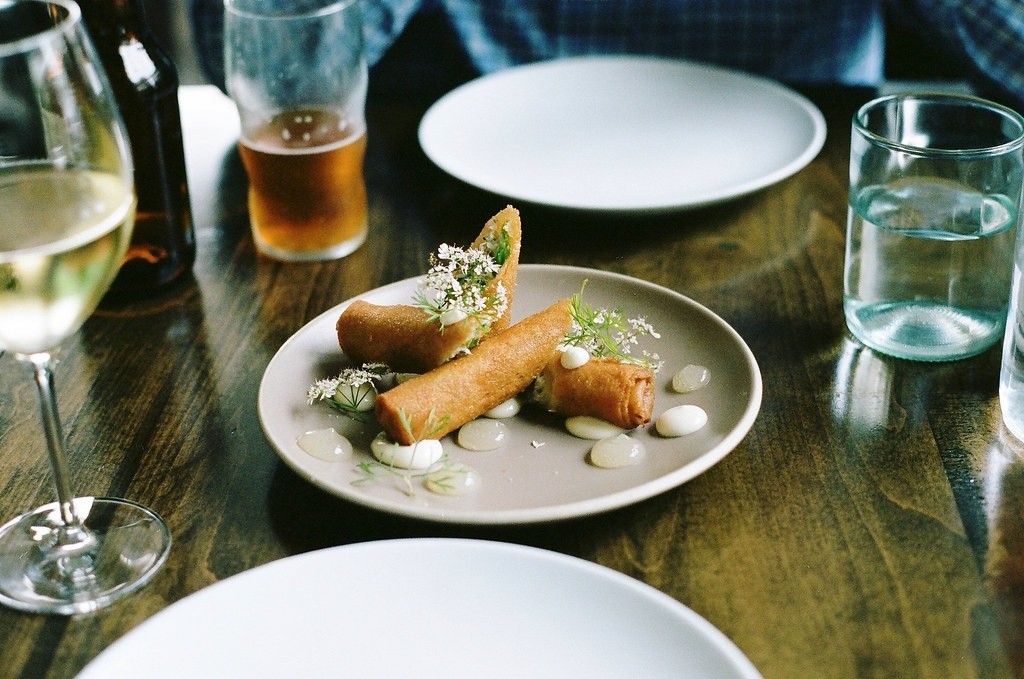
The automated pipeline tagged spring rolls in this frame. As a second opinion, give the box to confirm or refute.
[446,204,521,341]
[531,351,656,429]
[335,300,494,368]
[374,299,575,445]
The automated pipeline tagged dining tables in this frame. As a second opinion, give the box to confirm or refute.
[0,82,1024,679]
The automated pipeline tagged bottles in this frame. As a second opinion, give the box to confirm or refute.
[49,0,196,315]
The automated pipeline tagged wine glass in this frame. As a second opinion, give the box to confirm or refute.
[0,0,171,618]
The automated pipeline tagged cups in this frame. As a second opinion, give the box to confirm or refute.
[998,187,1024,443]
[221,0,371,264]
[842,91,1024,363]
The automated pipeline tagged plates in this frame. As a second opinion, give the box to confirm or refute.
[257,265,763,523]
[418,55,828,211]
[79,539,760,679]
